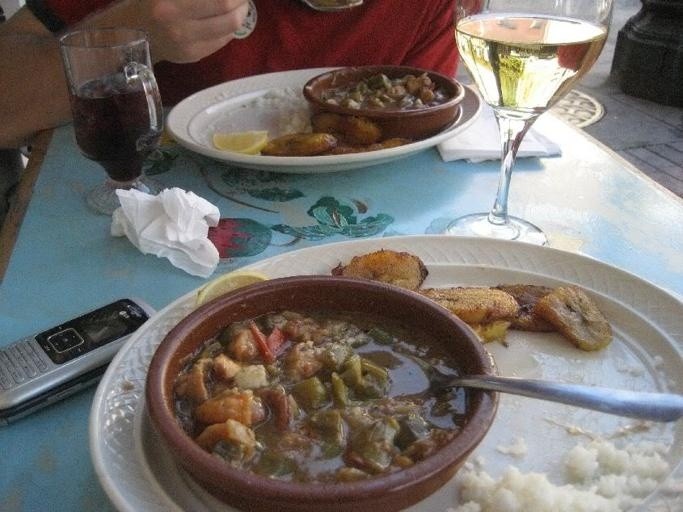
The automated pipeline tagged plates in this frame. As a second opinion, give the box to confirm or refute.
[85,233,682,512]
[165,63,484,175]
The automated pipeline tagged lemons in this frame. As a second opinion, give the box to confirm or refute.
[213,130,269,155]
[195,271,269,310]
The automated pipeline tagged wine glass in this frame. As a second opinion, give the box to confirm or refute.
[438,0,616,256]
[56,25,167,217]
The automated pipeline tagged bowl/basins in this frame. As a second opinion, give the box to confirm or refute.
[142,273,501,512]
[301,64,467,142]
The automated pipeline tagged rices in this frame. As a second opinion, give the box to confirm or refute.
[253,83,311,135]
[446,435,683,511]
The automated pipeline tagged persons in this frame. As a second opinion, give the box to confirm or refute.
[0,0,485,148]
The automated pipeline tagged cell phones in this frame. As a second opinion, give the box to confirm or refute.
[0,296,159,418]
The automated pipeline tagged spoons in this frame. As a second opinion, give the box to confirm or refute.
[389,353,683,423]
